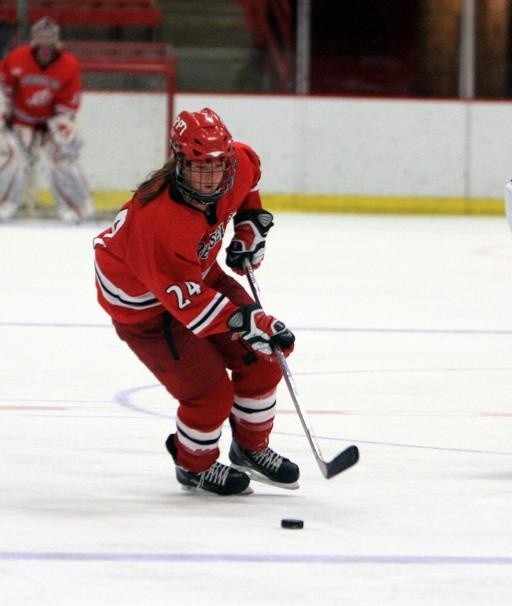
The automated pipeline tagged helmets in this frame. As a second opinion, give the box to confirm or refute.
[168,107,238,204]
[30,17,61,47]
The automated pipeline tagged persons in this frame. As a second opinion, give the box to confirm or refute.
[92,106,300,496]
[0,15,92,222]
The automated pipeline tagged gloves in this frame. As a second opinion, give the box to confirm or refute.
[225,207,275,277]
[225,302,296,362]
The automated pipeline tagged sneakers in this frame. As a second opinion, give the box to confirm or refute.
[175,461,251,496]
[228,440,301,484]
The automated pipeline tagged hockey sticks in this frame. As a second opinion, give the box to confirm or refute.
[242,258,356,478]
